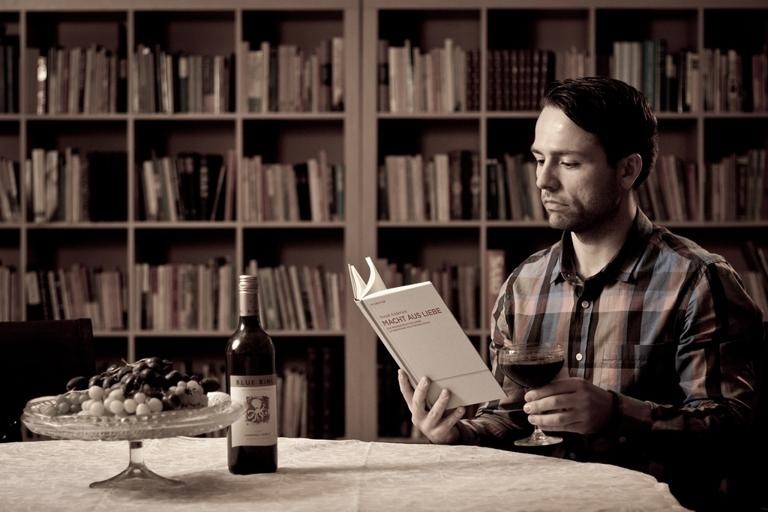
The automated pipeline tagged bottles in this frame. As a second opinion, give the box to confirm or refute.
[226,276,277,474]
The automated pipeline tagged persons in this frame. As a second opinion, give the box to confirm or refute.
[396,78,767,511]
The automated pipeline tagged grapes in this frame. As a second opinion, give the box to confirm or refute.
[42,355,221,417]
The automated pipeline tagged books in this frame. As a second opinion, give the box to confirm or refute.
[612,39,764,312]
[378,152,547,223]
[1,254,342,332]
[1,39,343,114]
[175,363,306,439]
[1,146,344,223]
[378,39,588,114]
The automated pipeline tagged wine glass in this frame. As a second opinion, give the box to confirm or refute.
[498,342,566,447]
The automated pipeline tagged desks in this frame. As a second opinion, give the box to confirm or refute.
[2,437,690,512]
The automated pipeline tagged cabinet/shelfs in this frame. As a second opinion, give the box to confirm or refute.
[360,3,767,442]
[1,1,365,437]
